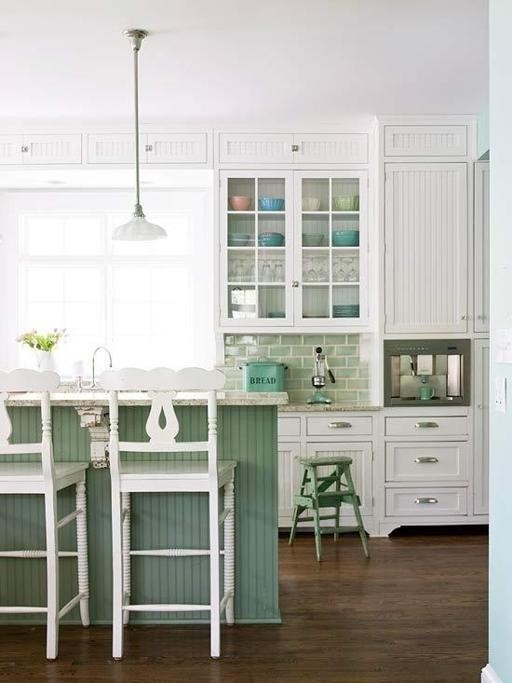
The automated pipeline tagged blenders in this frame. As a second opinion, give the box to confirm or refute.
[306,346,336,404]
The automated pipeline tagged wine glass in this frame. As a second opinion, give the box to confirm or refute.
[346,256,359,282]
[307,256,317,281]
[334,256,346,282]
[318,255,329,283]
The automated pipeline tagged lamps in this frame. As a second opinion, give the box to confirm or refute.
[113,26,166,241]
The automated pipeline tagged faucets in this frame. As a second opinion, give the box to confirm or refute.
[93,347,113,384]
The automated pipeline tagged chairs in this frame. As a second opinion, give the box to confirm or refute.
[1,369,91,659]
[100,367,237,662]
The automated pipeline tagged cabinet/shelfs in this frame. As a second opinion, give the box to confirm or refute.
[277,412,303,522]
[383,124,467,332]
[1,135,83,166]
[303,412,373,516]
[219,132,367,327]
[88,134,206,163]
[384,413,470,518]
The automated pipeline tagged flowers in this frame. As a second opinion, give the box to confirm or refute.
[14,327,69,352]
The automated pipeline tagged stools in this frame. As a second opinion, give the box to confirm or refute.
[288,457,370,562]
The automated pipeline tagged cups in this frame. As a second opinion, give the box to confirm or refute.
[262,263,273,281]
[234,264,245,282]
[249,264,255,282]
[274,264,284,282]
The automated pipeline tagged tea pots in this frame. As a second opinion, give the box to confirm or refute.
[416,378,435,401]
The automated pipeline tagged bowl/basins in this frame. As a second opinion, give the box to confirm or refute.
[302,233,325,247]
[229,196,252,210]
[332,230,359,246]
[334,195,359,211]
[303,198,322,211]
[259,232,284,247]
[258,197,284,210]
[228,233,250,246]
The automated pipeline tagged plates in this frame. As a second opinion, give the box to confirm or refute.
[332,304,358,318]
[303,315,329,318]
[269,312,285,317]
